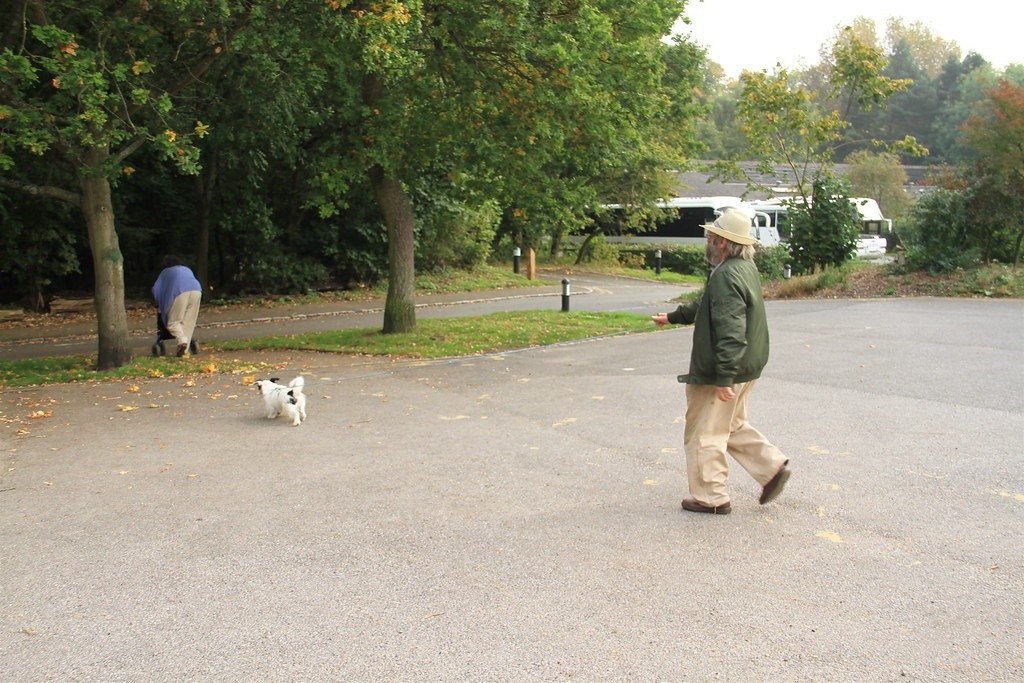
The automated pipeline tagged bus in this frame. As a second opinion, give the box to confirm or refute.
[543,196,777,249]
[754,197,893,260]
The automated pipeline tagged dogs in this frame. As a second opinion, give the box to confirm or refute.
[253,376,307,426]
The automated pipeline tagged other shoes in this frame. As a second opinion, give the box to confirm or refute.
[760,465,790,505]
[683,498,732,514]
[176,343,187,356]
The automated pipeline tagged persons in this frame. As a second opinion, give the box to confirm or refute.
[152,264,202,357]
[650,208,791,514]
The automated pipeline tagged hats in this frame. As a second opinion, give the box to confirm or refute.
[699,210,757,246]
